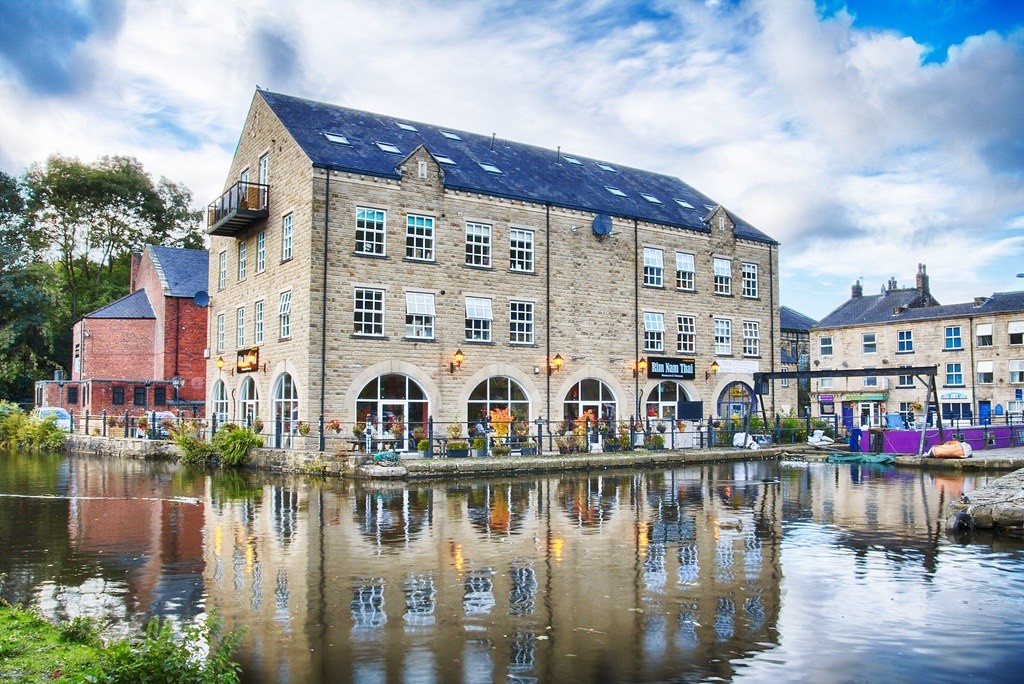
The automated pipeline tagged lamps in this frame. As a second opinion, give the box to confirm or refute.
[633,357,647,378]
[246,349,267,374]
[549,353,564,376]
[450,349,465,373]
[706,360,720,379]
[216,356,225,374]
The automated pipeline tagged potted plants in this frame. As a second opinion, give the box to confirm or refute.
[520,442,538,455]
[501,446,511,456]
[644,435,666,450]
[252,416,264,433]
[601,439,622,452]
[447,418,464,438]
[413,428,426,438]
[514,421,530,437]
[573,436,586,452]
[417,440,429,458]
[492,446,501,456]
[472,437,488,457]
[446,442,470,458]
[555,437,573,454]
[295,422,310,435]
[352,425,364,440]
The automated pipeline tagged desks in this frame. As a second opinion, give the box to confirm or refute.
[348,439,400,451]
[489,436,536,456]
[431,436,480,458]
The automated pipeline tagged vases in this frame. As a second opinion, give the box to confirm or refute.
[599,430,609,435]
[558,430,567,435]
[660,428,667,433]
[394,433,401,439]
[637,427,642,432]
[679,427,685,432]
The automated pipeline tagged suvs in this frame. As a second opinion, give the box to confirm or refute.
[135,411,180,440]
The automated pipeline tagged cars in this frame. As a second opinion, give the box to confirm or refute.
[35,407,74,432]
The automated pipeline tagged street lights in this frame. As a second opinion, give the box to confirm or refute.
[56,382,65,408]
[143,379,154,416]
[172,371,184,425]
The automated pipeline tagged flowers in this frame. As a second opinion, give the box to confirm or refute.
[553,422,568,431]
[598,422,610,432]
[656,418,668,430]
[325,420,342,433]
[390,416,406,433]
[677,420,686,427]
[635,422,643,428]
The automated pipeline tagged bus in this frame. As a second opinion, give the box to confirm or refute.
[18,403,34,413]
